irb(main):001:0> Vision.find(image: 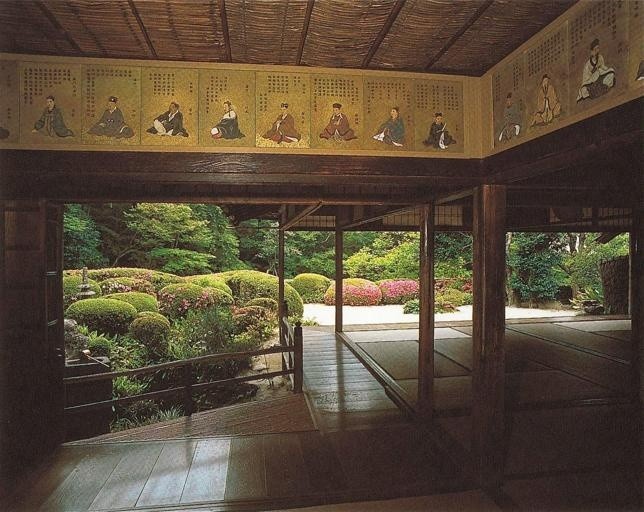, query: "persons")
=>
[495,93,522,143]
[421,111,457,148]
[87,95,133,139]
[372,107,405,148]
[209,101,245,139]
[575,37,615,104]
[258,103,302,143]
[143,102,190,138]
[529,74,561,125]
[29,96,73,138]
[318,103,358,141]
